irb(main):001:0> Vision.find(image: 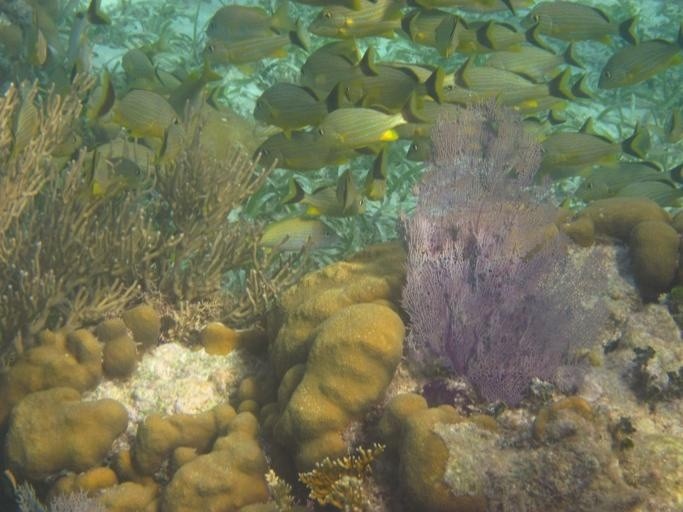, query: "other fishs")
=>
[0,0,682,254]
